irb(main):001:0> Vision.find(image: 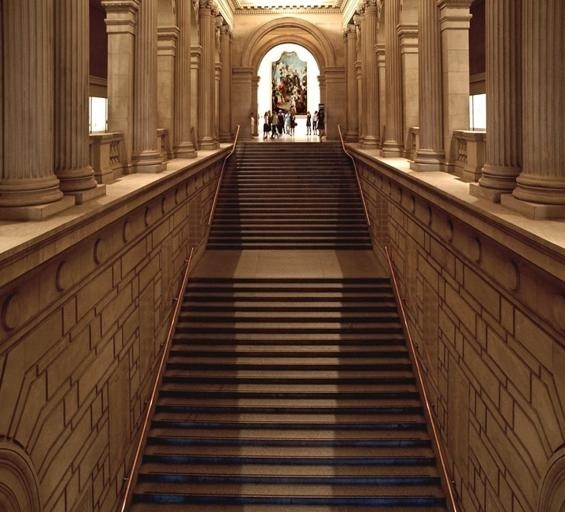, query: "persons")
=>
[272,65,307,112]
[251,104,324,139]
